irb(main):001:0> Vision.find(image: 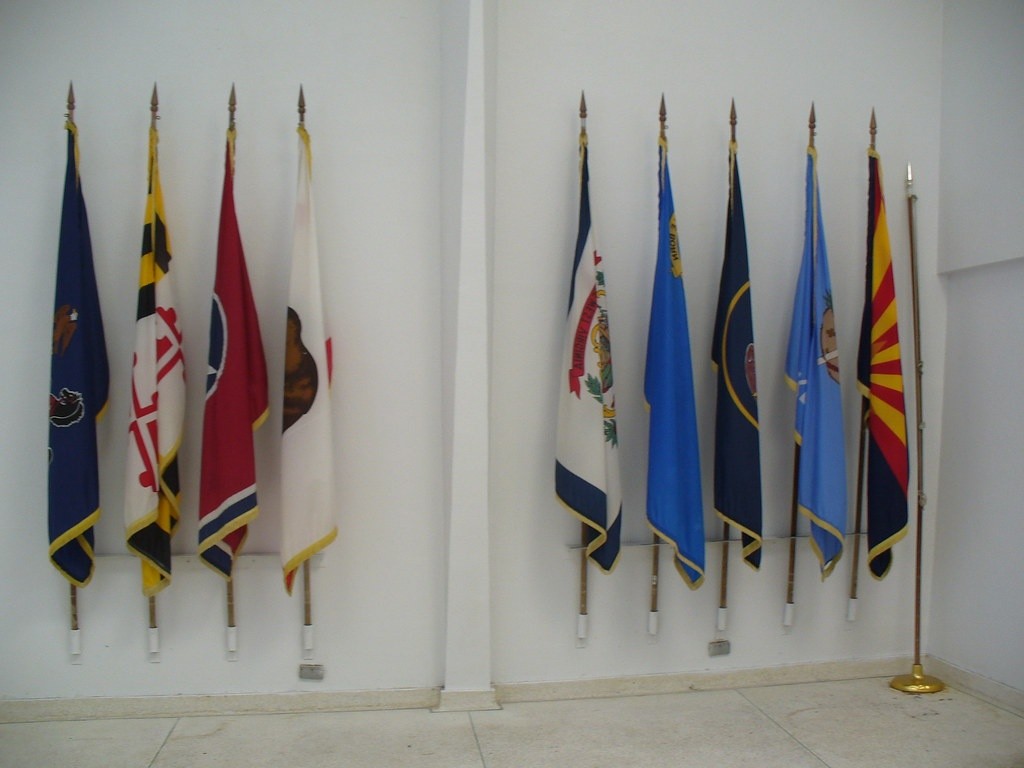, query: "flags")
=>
[699,143,766,576]
[45,116,111,589]
[122,128,186,598]
[779,147,847,582]
[199,131,271,585]
[642,139,707,590]
[281,129,338,596]
[554,144,625,577]
[862,149,910,582]
